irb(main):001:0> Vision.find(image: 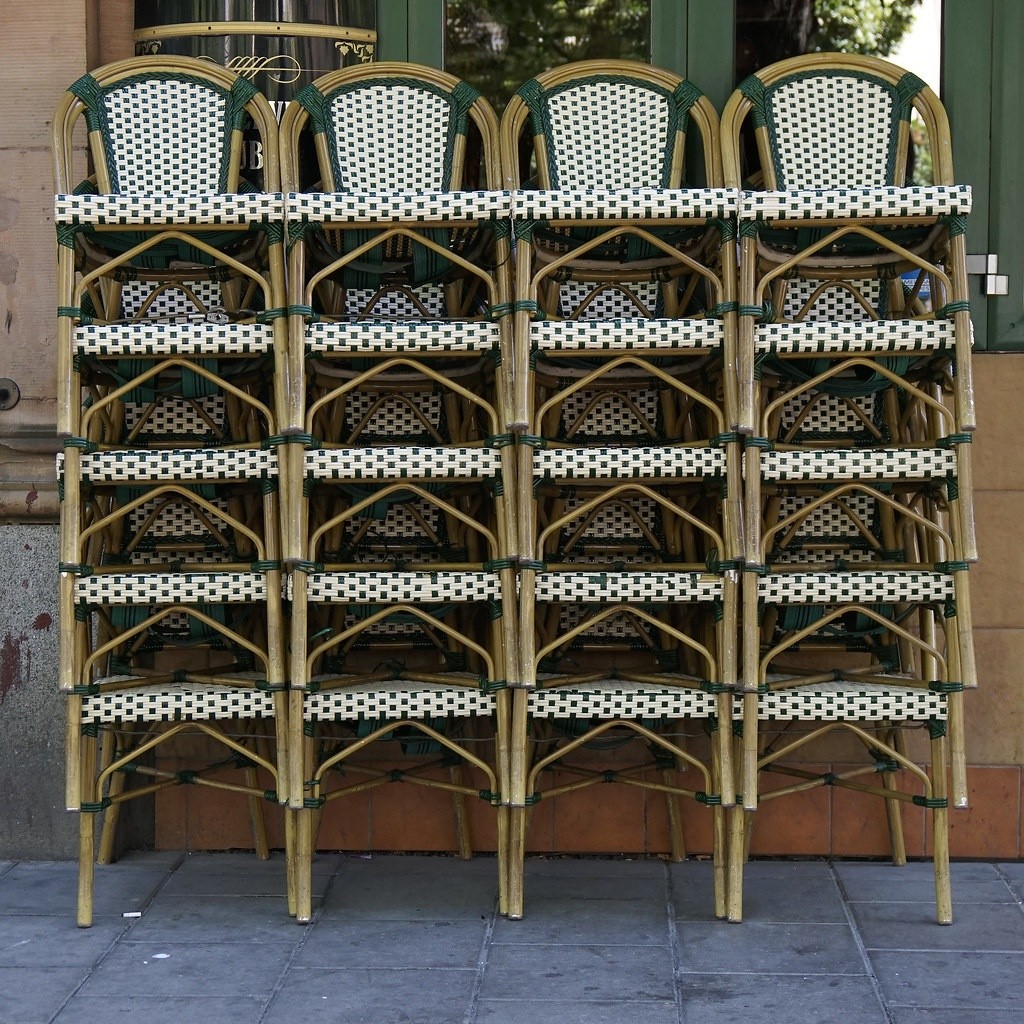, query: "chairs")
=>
[51,50,980,930]
